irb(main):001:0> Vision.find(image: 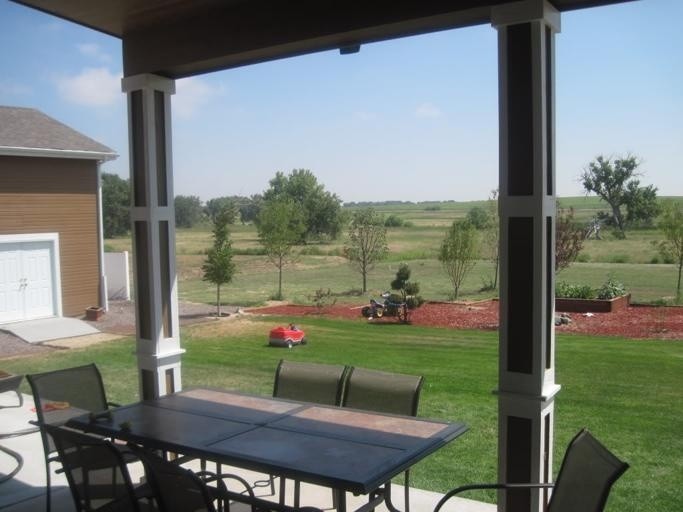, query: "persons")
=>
[287,322,298,332]
